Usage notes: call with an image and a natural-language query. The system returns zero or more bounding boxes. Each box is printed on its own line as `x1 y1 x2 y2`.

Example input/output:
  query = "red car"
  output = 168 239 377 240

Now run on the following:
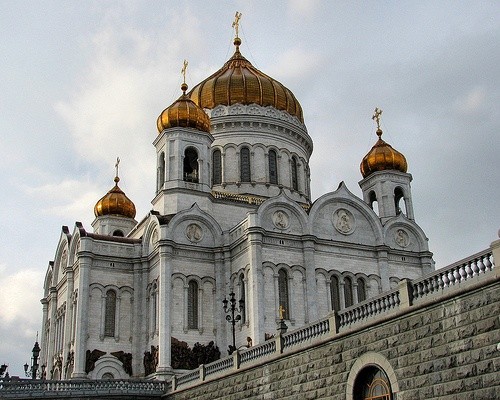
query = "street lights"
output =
222 290 244 355
0 364 10 381
24 342 47 380
